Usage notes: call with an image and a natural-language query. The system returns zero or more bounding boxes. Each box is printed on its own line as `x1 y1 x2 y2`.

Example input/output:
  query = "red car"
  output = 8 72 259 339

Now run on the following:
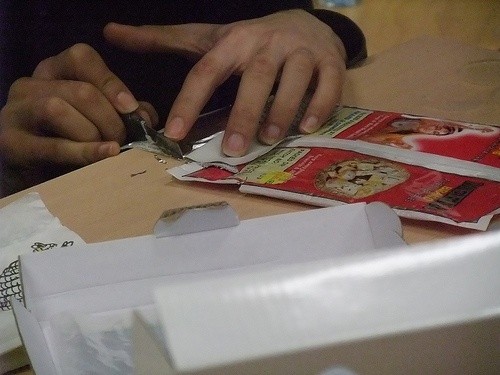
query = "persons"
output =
1 1 370 206
